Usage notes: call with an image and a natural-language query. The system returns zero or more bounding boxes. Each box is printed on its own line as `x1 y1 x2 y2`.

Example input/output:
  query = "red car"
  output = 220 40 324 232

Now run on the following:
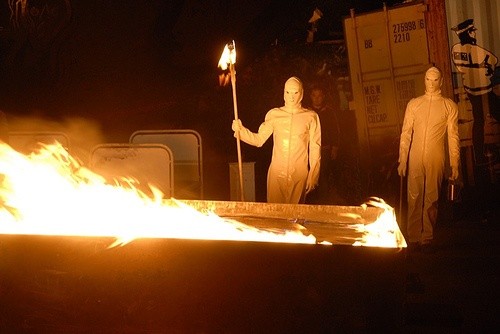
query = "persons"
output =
307 87 340 193
233 77 322 204
397 67 460 257
